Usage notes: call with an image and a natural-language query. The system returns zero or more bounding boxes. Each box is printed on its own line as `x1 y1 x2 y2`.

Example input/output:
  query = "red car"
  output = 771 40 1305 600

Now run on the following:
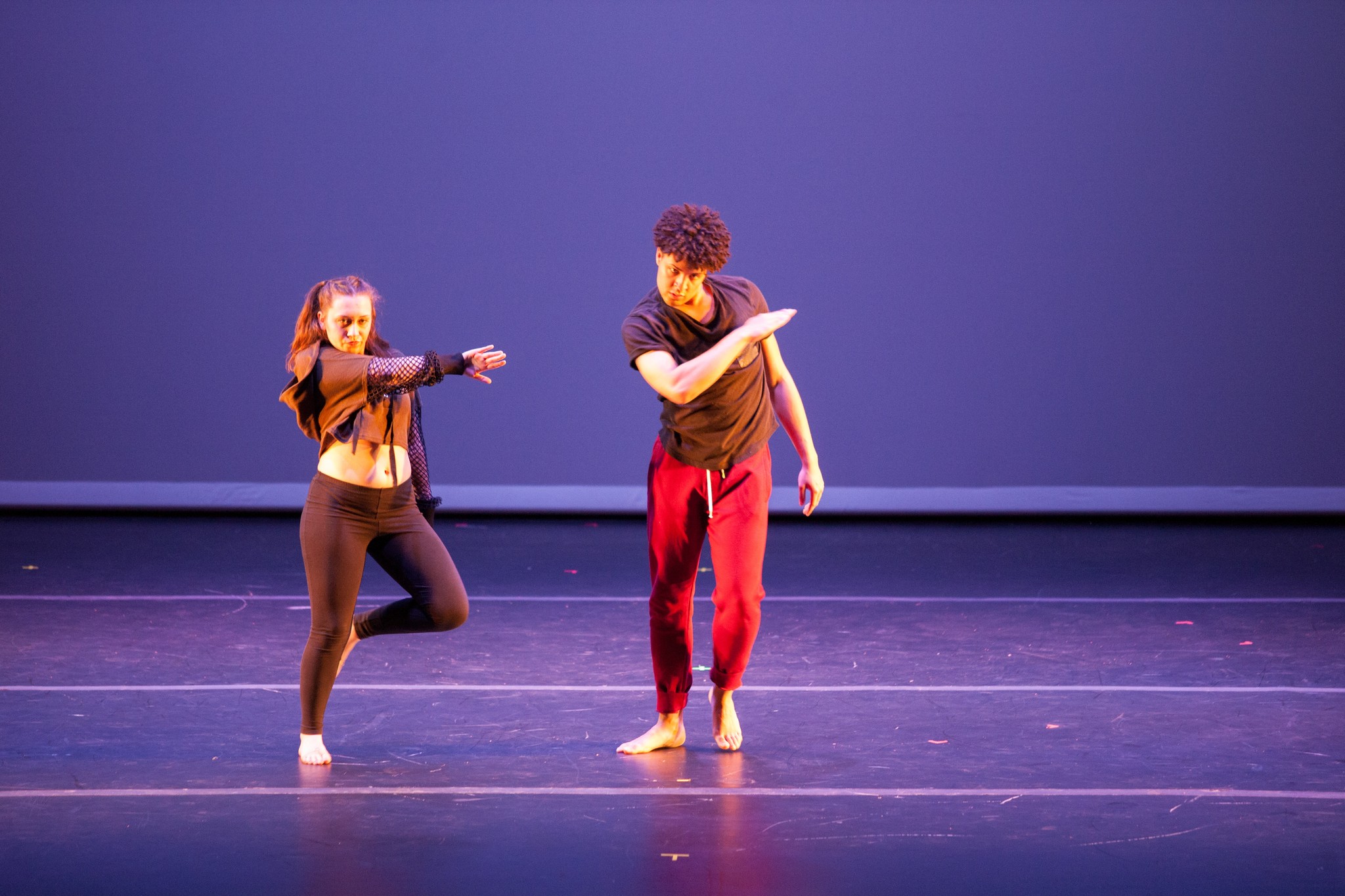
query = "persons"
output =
274 273 506 766
615 203 825 756
